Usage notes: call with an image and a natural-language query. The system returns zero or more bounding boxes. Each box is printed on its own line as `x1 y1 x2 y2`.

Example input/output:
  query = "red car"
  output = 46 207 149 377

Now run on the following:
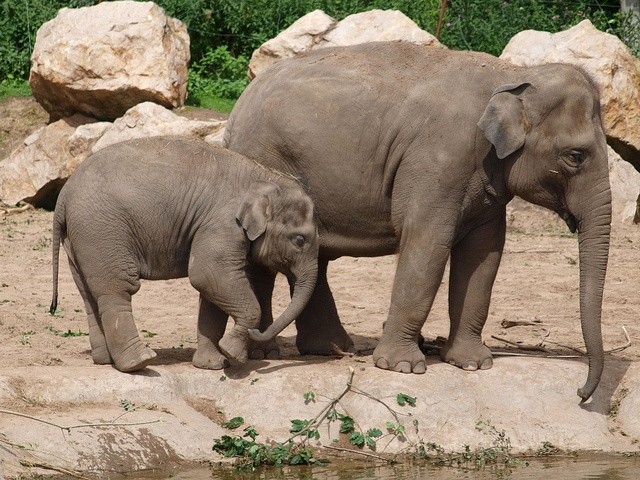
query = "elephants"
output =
49 136 320 373
220 40 611 401
